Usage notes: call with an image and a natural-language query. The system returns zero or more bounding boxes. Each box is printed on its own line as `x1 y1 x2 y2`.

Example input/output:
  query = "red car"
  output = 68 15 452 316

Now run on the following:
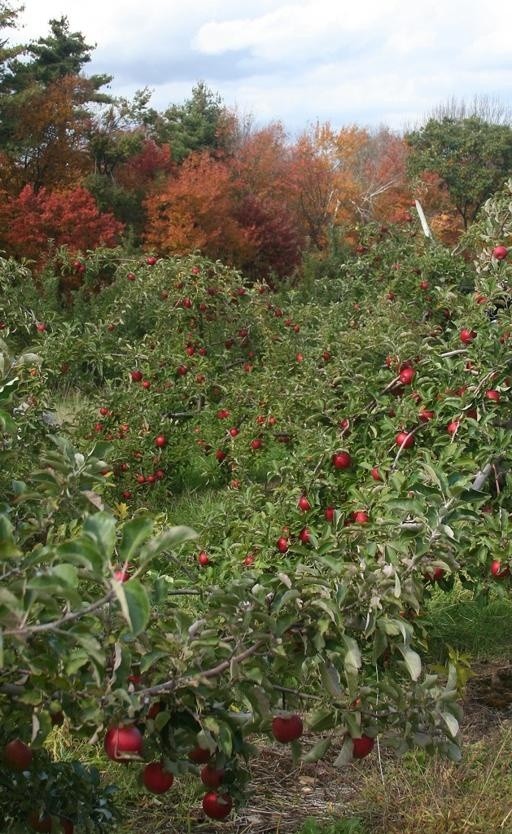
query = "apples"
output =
6 211 511 831
